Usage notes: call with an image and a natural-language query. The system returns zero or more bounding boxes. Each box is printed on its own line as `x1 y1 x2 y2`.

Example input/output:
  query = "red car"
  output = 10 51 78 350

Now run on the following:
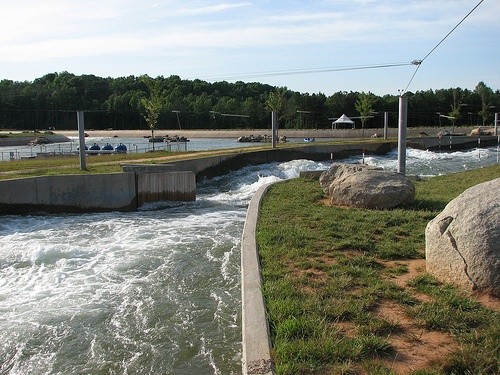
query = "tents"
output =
332 112 357 130
